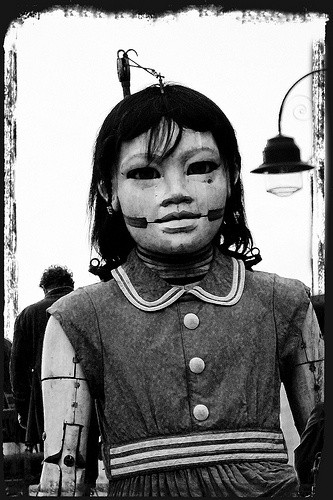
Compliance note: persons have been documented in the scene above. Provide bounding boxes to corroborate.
[8,266,78,453]
[36,81,325,499]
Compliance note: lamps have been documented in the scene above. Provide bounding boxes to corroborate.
[248,67,324,199]
